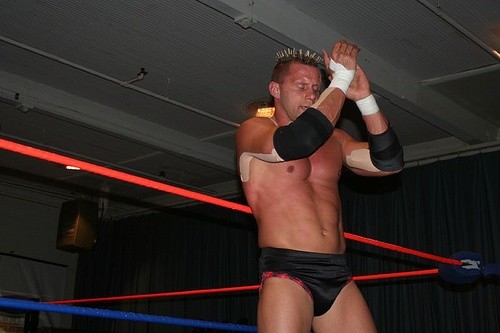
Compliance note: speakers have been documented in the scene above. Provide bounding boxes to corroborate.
[57,200,96,254]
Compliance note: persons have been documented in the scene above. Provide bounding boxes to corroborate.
[233,39,407,332]
